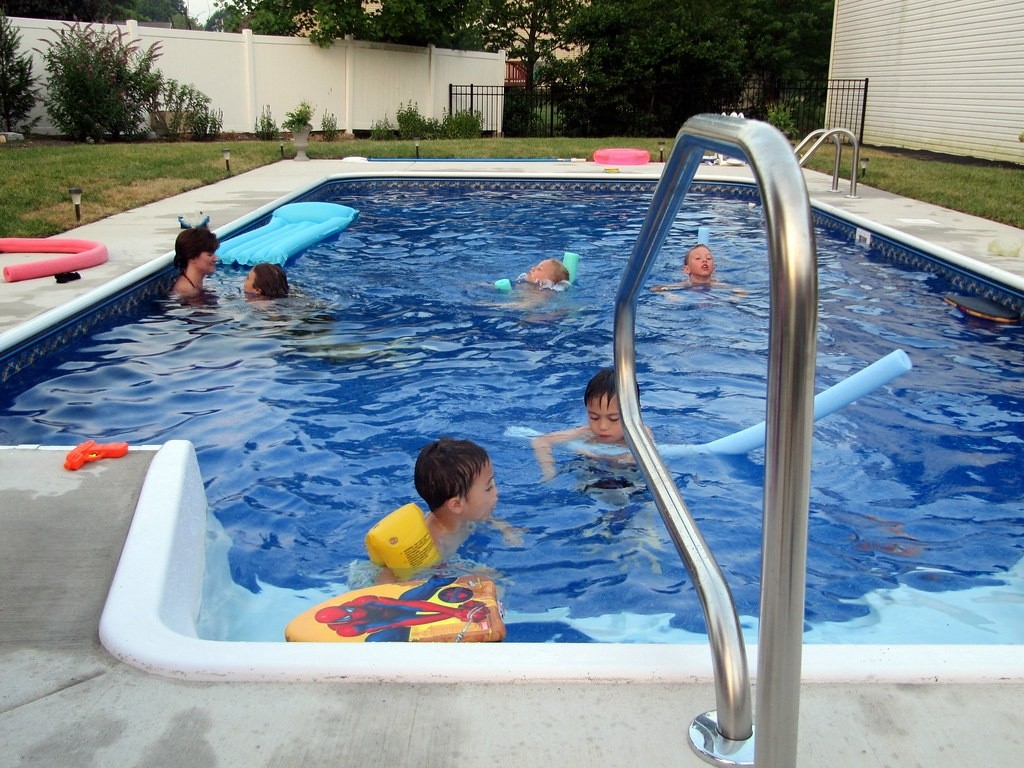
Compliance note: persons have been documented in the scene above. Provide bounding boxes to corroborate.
[170,229,220,293]
[473,258,569,310]
[531,368,655,483]
[244,261,290,295]
[376,439,524,587]
[653,244,748,305]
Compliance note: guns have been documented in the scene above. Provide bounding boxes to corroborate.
[64,440,128,471]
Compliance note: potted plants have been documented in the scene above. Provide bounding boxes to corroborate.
[768,103,799,138]
[283,103,315,161]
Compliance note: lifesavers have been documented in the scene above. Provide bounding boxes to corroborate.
[367,502,442,568]
[593,148,650,165]
[495,248,580,291]
[0,237,109,282]
[714,349,912,453]
[697,226,711,247]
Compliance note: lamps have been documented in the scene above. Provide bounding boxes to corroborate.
[278,137,283,146]
[221,149,231,160]
[860,157,869,168]
[68,189,82,205]
[658,142,665,151]
[414,137,420,146]
